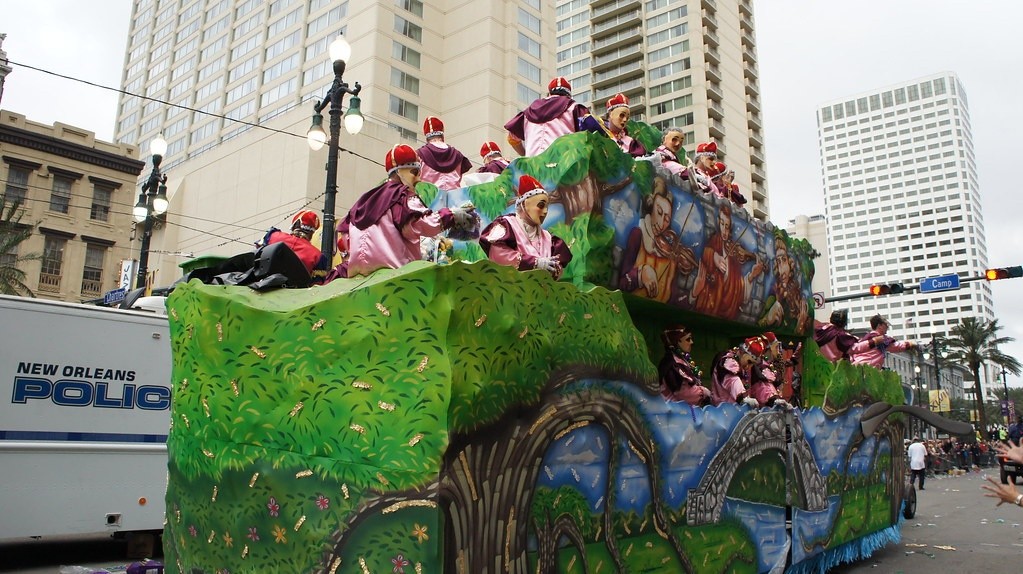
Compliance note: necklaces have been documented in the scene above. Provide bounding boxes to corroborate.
[676,350,702,377]
[764,355,778,376]
[732,352,750,390]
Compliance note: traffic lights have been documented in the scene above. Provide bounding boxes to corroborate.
[870,282,904,296]
[984,265,1023,281]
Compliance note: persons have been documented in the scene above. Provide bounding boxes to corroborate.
[848,313,913,370]
[904,439,911,456]
[920,437,969,476]
[964,439,996,469]
[980,416,1023,509]
[479,174,574,282]
[658,322,711,406]
[714,162,748,205]
[338,142,477,277]
[816,307,862,361]
[908,436,927,490]
[415,116,472,191]
[600,92,646,156]
[749,332,784,408]
[504,77,610,158]
[690,141,727,197]
[474,141,511,175]
[711,336,767,408]
[727,168,739,191]
[650,127,708,186]
[255,209,329,286]
[787,338,802,406]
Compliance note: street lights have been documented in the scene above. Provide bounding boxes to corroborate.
[914,365,924,441]
[922,333,949,440]
[306,27,366,275]
[132,131,169,297]
[995,365,1010,425]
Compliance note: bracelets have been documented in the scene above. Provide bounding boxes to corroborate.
[1015,493,1022,506]
